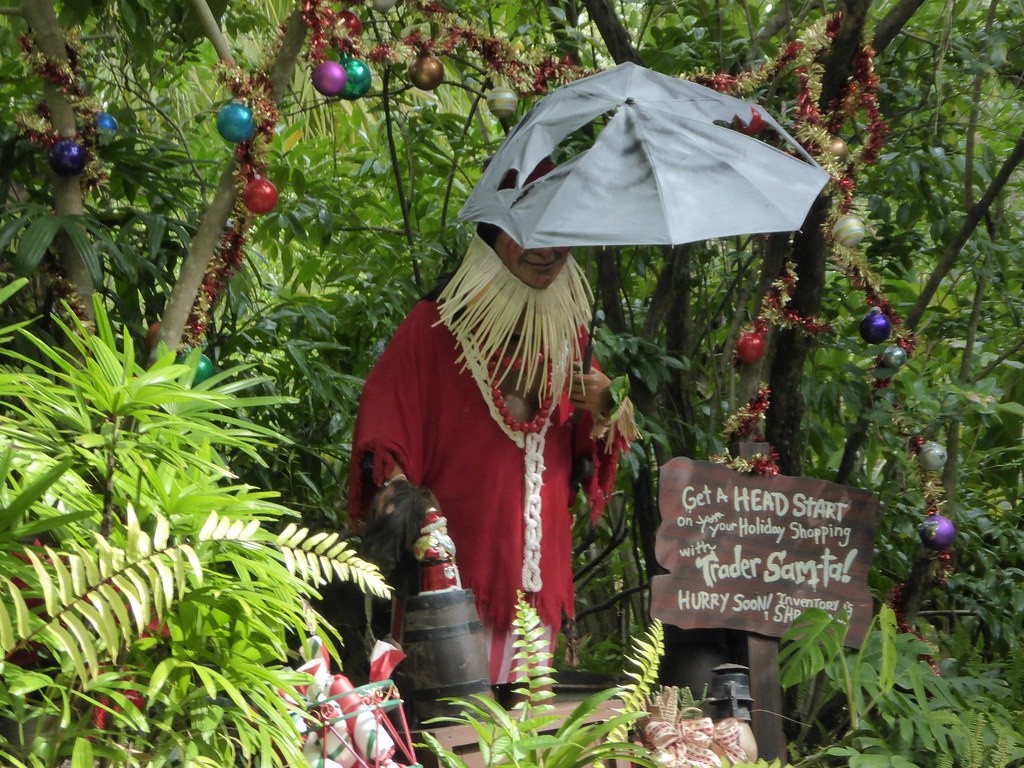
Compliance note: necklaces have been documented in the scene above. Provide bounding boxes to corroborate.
[468,325,553,432]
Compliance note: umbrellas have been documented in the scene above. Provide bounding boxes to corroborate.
[457,62,829,423]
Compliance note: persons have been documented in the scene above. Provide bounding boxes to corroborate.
[346,155,635,690]
